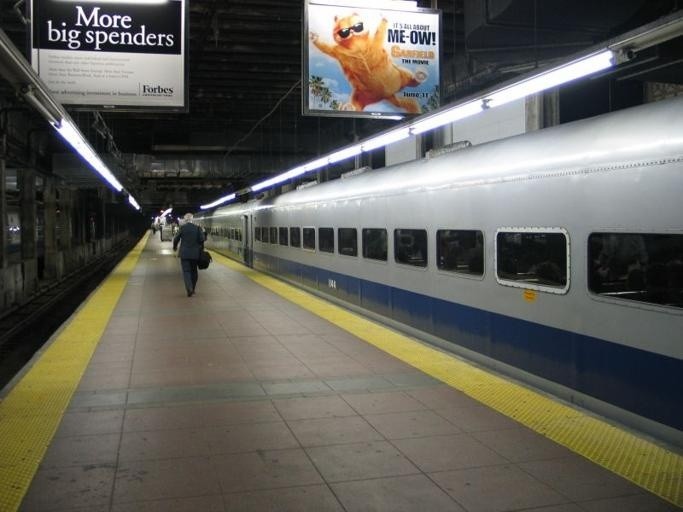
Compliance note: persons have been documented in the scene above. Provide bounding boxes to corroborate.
[173,213,205,296]
[588,234,682,306]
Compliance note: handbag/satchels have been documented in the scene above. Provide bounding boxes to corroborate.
[196,248,212,270]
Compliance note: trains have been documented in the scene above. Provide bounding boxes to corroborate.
[182,97,682,456]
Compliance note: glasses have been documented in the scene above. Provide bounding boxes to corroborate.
[337,23,363,38]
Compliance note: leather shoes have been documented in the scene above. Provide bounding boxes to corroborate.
[187,288,192,296]
